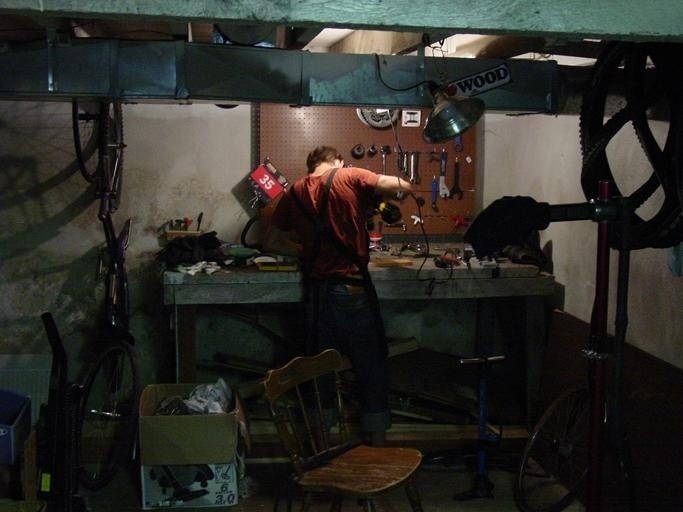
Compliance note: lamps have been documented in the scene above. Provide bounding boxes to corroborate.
[373,51,484,145]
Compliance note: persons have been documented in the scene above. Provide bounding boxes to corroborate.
[271,147,414,449]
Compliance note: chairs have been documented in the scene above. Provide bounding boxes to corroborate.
[259,349,423,512]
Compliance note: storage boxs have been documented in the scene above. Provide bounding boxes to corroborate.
[133,383,247,512]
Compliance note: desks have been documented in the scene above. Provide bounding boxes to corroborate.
[163,241,555,436]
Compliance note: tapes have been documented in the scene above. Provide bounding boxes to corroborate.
[352,144,366,159]
